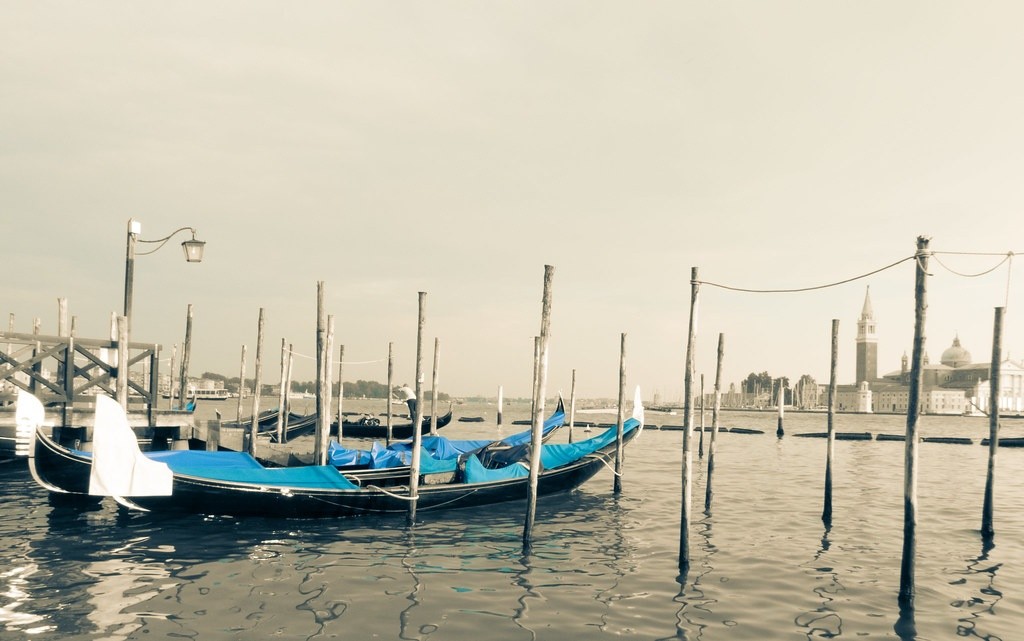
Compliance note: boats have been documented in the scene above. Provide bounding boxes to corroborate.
[31,394,565,495]
[643,406,677,415]
[211,406,317,445]
[162,388,229,401]
[96,385,644,517]
[328,403,453,440]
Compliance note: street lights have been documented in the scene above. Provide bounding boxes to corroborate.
[124,217,206,411]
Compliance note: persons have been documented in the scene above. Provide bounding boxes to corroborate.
[394,383,416,423]
